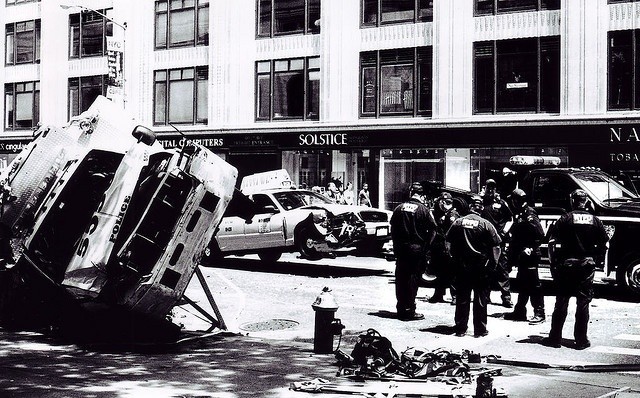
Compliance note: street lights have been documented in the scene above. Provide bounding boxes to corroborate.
[59,4,127,109]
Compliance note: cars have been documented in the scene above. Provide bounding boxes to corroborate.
[201,188,394,266]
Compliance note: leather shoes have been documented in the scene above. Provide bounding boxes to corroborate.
[528,316,546,325]
[504,312,527,320]
[450,325,468,334]
[406,311,425,320]
[428,294,443,303]
[503,300,514,308]
[474,329,489,337]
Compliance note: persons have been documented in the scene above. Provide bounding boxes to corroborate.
[326,178,341,204]
[342,182,356,207]
[428,190,455,304]
[391,185,436,312]
[475,178,514,308]
[444,196,500,314]
[546,190,607,323]
[356,180,374,208]
[505,189,543,322]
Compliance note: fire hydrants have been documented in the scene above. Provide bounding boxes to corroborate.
[311,286,344,354]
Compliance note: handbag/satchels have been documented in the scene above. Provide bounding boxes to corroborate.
[447,260,465,291]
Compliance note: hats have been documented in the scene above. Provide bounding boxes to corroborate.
[471,195,483,205]
[432,194,452,203]
[507,188,526,199]
[486,178,496,184]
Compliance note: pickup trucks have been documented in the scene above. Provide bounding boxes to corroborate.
[420,155,640,297]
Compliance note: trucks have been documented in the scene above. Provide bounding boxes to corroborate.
[1,96,238,337]
